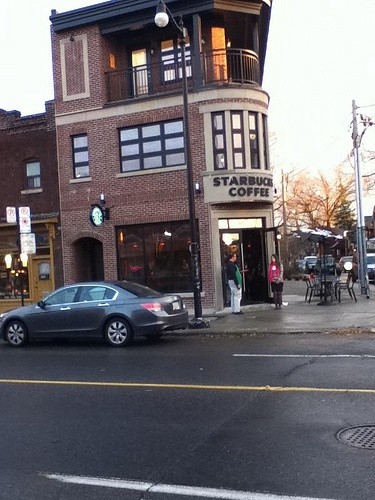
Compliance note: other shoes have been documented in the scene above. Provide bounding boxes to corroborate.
[232,311,244,315]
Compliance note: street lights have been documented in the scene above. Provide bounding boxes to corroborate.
[4,253,29,306]
[348,98,370,295]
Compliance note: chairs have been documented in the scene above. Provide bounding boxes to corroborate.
[303,271,358,304]
[79,290,93,301]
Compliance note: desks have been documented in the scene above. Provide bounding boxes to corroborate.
[316,278,340,307]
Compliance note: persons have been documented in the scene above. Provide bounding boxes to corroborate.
[268,254,284,310]
[226,252,245,316]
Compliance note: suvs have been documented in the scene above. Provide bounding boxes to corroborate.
[351,253,375,285]
[339,256,354,273]
[295,254,335,275]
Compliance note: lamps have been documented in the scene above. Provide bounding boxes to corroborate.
[100,190,107,205]
[195,182,201,194]
[276,228,282,240]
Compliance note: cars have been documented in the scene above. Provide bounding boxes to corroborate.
[0,281,188,348]
